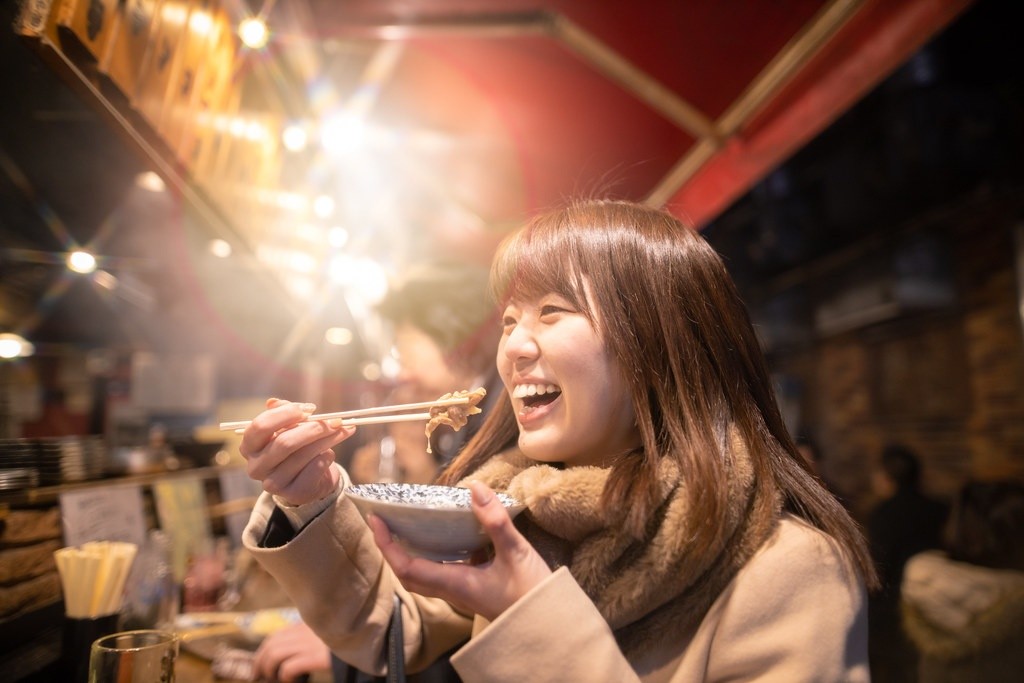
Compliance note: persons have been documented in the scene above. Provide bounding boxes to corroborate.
[237,199,879,683]
[179,264,499,683]
[789,436,1024,683]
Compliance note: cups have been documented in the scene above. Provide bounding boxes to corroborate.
[60,613,183,683]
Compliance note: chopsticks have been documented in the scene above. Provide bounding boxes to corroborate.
[220,396,467,435]
[54,541,137,618]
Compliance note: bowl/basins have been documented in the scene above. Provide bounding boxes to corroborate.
[112,446,161,473]
[345,483,528,562]
[173,443,223,468]
[167,609,303,661]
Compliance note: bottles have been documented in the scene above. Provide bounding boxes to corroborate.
[118,529,174,631]
[182,535,217,613]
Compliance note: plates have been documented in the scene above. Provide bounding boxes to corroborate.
[0,431,114,491]
